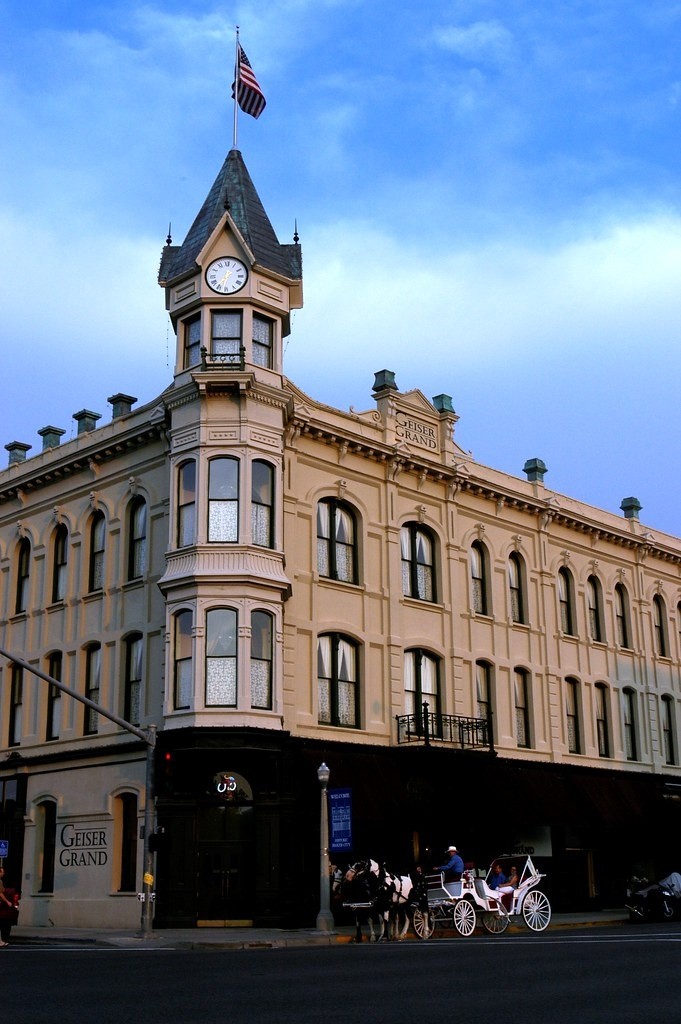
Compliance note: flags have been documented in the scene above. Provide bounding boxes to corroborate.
[327,787,353,852]
[231,41,267,121]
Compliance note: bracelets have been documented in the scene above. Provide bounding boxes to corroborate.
[504,884,505,886]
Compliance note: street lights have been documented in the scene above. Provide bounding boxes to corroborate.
[316,762,335,931]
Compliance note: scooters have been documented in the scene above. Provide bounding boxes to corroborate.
[628,880,676,921]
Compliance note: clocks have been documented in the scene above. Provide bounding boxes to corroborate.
[204,256,249,296]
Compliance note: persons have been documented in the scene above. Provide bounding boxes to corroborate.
[332,865,343,894]
[0,867,13,947]
[488,863,519,897]
[433,845,464,884]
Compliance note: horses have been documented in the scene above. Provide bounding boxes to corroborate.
[331,856,429,942]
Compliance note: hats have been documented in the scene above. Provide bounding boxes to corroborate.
[446,846,458,852]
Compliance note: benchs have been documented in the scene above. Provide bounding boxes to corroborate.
[444,862,474,896]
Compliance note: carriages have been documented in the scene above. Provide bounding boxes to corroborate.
[332,852,552,945]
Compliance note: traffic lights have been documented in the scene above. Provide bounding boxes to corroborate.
[155,741,175,781]
